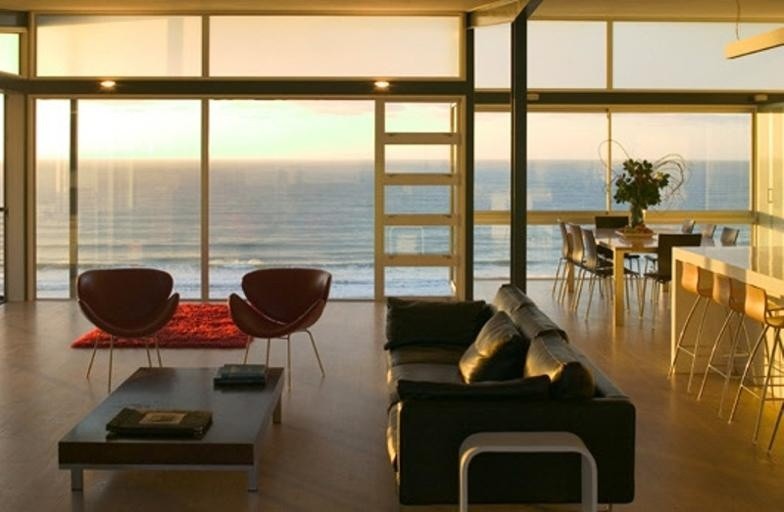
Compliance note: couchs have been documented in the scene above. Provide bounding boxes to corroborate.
[381,281,637,510]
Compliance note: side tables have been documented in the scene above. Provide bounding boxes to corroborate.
[458,433,598,509]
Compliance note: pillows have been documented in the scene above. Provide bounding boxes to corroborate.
[394,374,549,400]
[456,310,526,383]
[383,296,488,350]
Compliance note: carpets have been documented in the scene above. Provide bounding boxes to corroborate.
[70,302,254,349]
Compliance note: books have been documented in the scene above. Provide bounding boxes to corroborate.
[106,408,211,436]
[213,365,269,386]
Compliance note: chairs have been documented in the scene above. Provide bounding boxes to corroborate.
[229,265,332,391]
[76,268,179,393]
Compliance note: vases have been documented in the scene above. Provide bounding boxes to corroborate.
[598,138,688,209]
[631,207,644,224]
